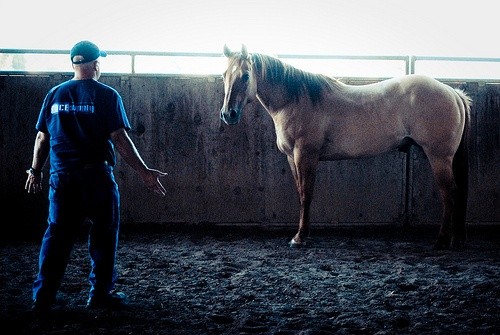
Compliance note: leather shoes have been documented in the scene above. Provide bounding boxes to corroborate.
[29,299,53,312]
[86,290,127,310]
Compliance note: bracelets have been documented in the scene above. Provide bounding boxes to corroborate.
[28,167,42,175]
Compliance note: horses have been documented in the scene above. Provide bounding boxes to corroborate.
[220,43,473,251]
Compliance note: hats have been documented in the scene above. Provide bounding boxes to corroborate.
[69,40,107,66]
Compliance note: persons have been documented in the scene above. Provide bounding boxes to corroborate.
[24,39,168,312]
